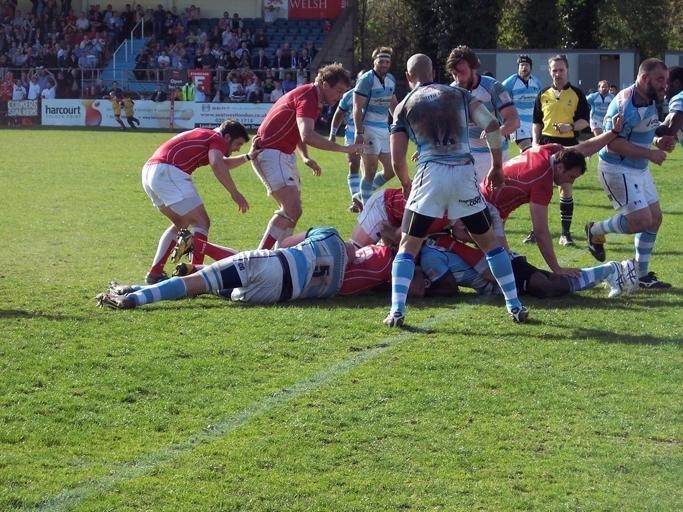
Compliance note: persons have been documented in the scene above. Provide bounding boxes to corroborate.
[103,43,683,329]
[323,19,332,33]
[2,1,318,103]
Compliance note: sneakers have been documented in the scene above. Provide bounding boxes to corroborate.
[585,221,605,262]
[559,234,575,246]
[350,193,363,212]
[382,311,405,327]
[96,280,136,310]
[524,230,536,243]
[145,272,169,284]
[172,262,193,277]
[171,228,193,263]
[512,306,529,323]
[605,258,640,298]
[639,271,671,289]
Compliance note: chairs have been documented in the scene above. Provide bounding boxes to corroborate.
[184,16,336,67]
